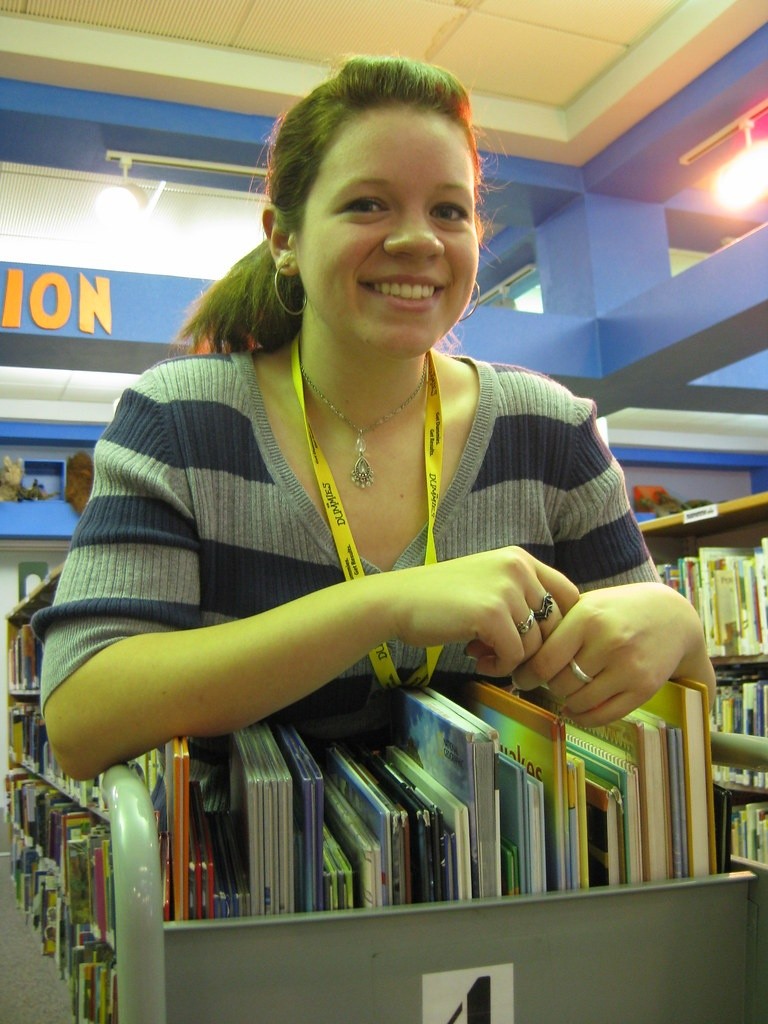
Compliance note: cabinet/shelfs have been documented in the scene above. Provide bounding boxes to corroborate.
[4,561,163,1023]
[637,491,768,868]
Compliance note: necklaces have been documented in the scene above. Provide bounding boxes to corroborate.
[301,358,428,489]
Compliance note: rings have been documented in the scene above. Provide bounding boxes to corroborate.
[534,594,553,621]
[570,659,593,684]
[517,609,536,635]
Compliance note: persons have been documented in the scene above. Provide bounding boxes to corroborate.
[33,56,717,780]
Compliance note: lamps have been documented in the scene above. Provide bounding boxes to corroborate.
[93,155,147,219]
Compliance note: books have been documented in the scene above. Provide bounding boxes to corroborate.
[653,536,768,660]
[709,672,768,873]
[171,680,718,921]
[8,623,44,689]
[10,704,115,1024]
